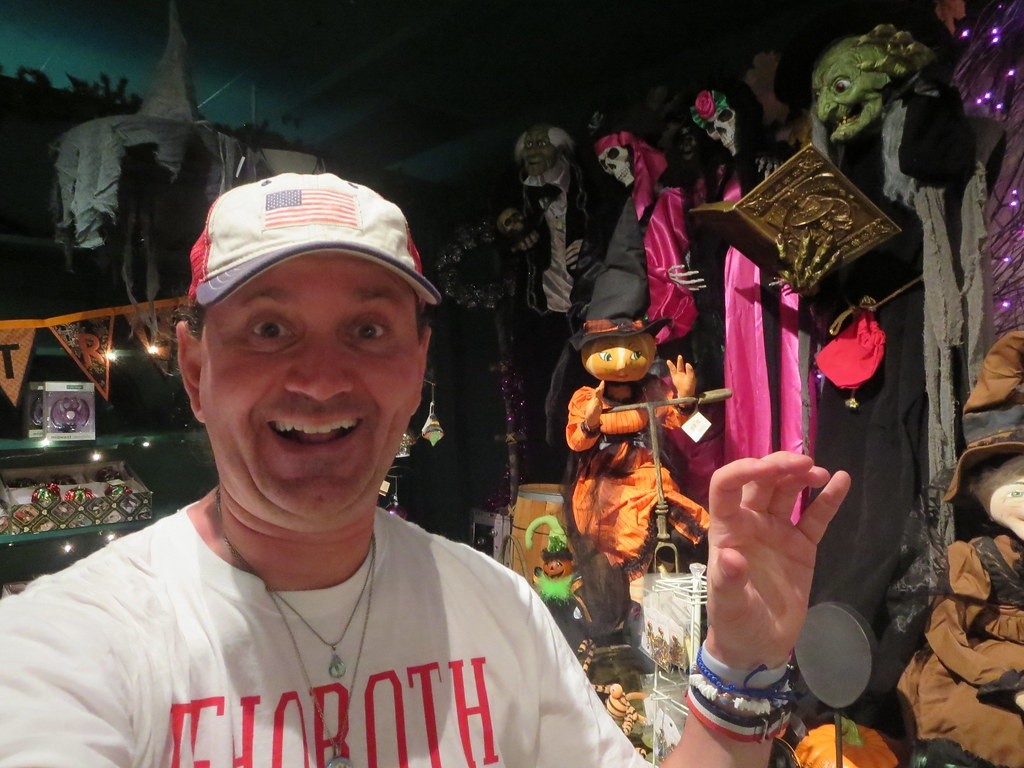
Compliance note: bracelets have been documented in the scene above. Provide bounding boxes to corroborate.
[684,639,794,743]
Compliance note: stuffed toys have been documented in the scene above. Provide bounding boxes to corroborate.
[896,332,1024,768]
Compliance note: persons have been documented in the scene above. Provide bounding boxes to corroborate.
[564,198,710,582]
[0,171,853,768]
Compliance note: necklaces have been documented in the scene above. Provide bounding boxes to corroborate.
[216,486,375,768]
[217,487,375,677]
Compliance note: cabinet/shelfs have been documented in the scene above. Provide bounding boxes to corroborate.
[0,346,207,543]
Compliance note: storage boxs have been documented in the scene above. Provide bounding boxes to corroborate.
[41,382,96,441]
[0,460,153,534]
[22,380,43,439]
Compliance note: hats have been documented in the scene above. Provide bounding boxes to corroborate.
[188,172,442,308]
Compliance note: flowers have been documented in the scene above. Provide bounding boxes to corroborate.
[694,90,716,119]
